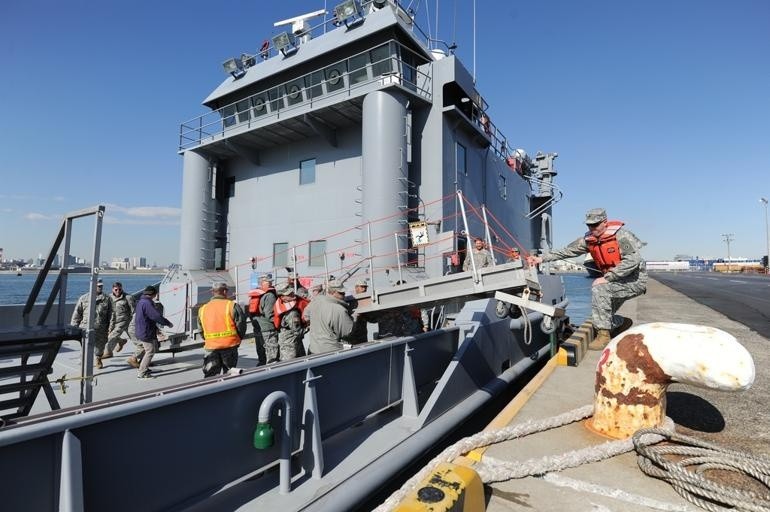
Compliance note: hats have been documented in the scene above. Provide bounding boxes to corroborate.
[97,278,103,287]
[143,286,157,295]
[585,208,607,224]
[213,272,369,296]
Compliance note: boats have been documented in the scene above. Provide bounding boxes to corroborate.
[583,253,603,277]
[16,273,22,277]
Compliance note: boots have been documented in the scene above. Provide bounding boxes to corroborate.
[588,317,632,350]
[96,339,156,380]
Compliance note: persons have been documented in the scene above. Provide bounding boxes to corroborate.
[198,271,429,378]
[70,278,174,378]
[462,237,523,272]
[524,207,649,350]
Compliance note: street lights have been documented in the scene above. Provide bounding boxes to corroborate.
[759,198,770,269]
[721,232,736,273]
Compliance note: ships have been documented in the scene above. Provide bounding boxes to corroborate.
[0,0,572,512]
